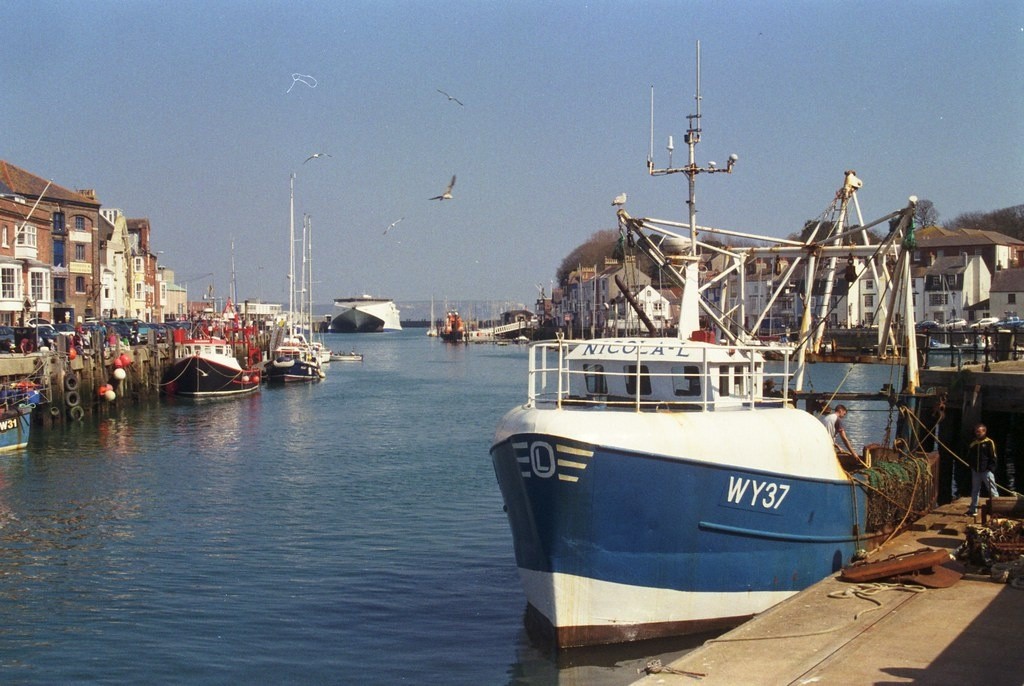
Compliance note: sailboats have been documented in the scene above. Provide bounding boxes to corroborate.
[263,172,331,401]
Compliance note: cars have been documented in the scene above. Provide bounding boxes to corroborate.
[894,313,1024,331]
[0,317,210,350]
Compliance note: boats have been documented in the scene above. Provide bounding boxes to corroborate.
[0,347,53,453]
[425,293,529,346]
[488,38,946,650]
[334,294,403,333]
[333,353,364,362]
[162,341,263,401]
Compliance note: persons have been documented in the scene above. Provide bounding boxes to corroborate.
[241,317,245,326]
[828,319,832,329]
[838,319,847,327]
[823,405,859,461]
[252,318,256,323]
[964,423,1000,516]
[74,322,83,336]
[274,318,284,328]
[0,338,15,353]
[856,320,859,326]
[862,319,864,327]
[99,323,108,347]
[16,337,34,356]
[81,329,91,346]
[132,321,139,345]
[109,333,117,347]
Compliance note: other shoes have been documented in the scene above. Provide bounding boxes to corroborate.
[964,511,978,517]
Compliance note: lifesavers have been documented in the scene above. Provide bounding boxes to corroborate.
[64,373,85,421]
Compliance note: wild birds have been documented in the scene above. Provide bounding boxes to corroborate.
[611,192,628,207]
[383,217,404,235]
[438,88,464,108]
[427,175,456,201]
[303,151,332,163]
